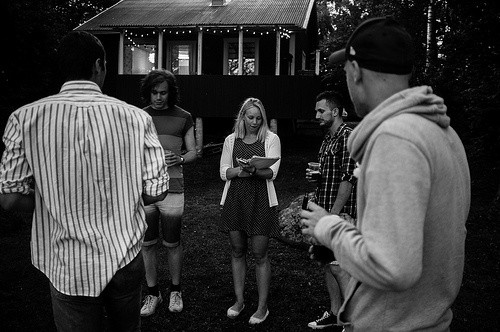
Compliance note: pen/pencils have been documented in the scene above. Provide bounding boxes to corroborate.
[302,192,309,210]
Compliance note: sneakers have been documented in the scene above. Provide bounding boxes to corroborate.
[307,310,342,329]
[140,290,163,317]
[168,290,183,312]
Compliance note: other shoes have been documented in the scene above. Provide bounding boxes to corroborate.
[226,302,245,318]
[248,308,269,324]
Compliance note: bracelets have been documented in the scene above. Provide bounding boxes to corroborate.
[180,155,184,162]
[249,167,256,176]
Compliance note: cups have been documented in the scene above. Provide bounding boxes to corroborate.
[308,161,321,182]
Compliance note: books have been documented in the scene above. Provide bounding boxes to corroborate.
[238,155,279,177]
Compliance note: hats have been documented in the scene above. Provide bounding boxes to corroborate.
[329,14,414,77]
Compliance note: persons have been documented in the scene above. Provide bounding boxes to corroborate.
[0,31,169,332]
[299,14,470,332]
[220,97,281,324]
[306,92,358,332]
[141,70,197,317]
